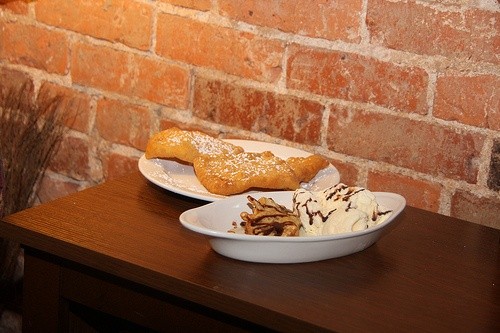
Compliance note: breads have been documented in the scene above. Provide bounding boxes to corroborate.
[287,153,330,184]
[145,126,243,163]
[194,149,300,194]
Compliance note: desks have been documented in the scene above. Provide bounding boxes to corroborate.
[0,159,500,333]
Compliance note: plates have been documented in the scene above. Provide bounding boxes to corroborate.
[179,190,407,263]
[138,138,341,202]
